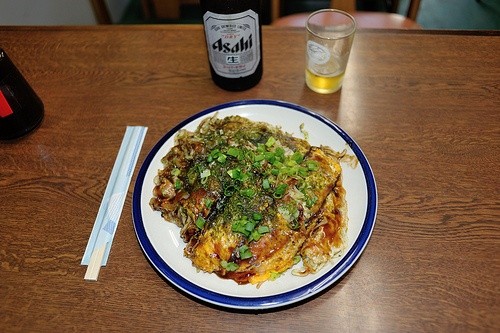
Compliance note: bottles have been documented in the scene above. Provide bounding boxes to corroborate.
[201,0,263,91]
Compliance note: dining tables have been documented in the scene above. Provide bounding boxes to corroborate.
[0,24,500,332]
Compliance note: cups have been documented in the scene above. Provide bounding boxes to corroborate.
[304,9,356,95]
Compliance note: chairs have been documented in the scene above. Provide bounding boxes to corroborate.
[271,0,421,26]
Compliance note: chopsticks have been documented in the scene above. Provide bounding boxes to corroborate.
[84,126,141,280]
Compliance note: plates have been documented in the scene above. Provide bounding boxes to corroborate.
[131,100,379,309]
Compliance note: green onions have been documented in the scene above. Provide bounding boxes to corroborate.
[178,128,320,259]
